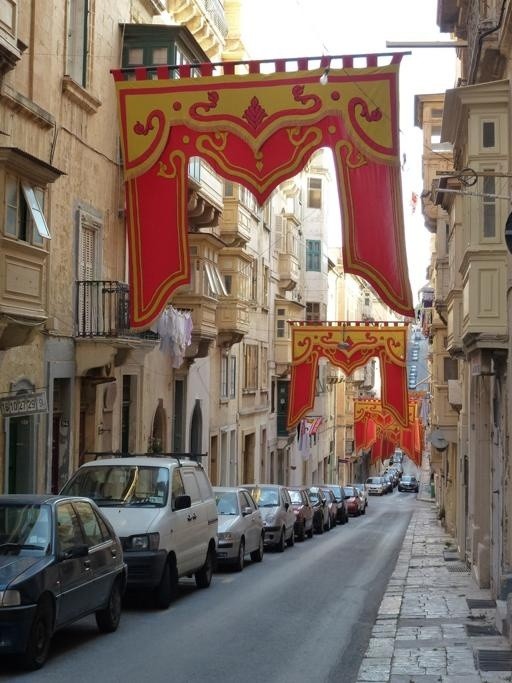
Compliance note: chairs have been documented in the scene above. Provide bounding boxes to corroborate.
[100,468,122,499]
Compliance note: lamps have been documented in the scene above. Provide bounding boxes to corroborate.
[98,422,112,435]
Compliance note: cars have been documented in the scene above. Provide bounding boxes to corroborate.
[364,451,419,496]
[0,489,131,675]
[210,482,368,575]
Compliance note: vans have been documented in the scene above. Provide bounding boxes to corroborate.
[52,450,221,616]
